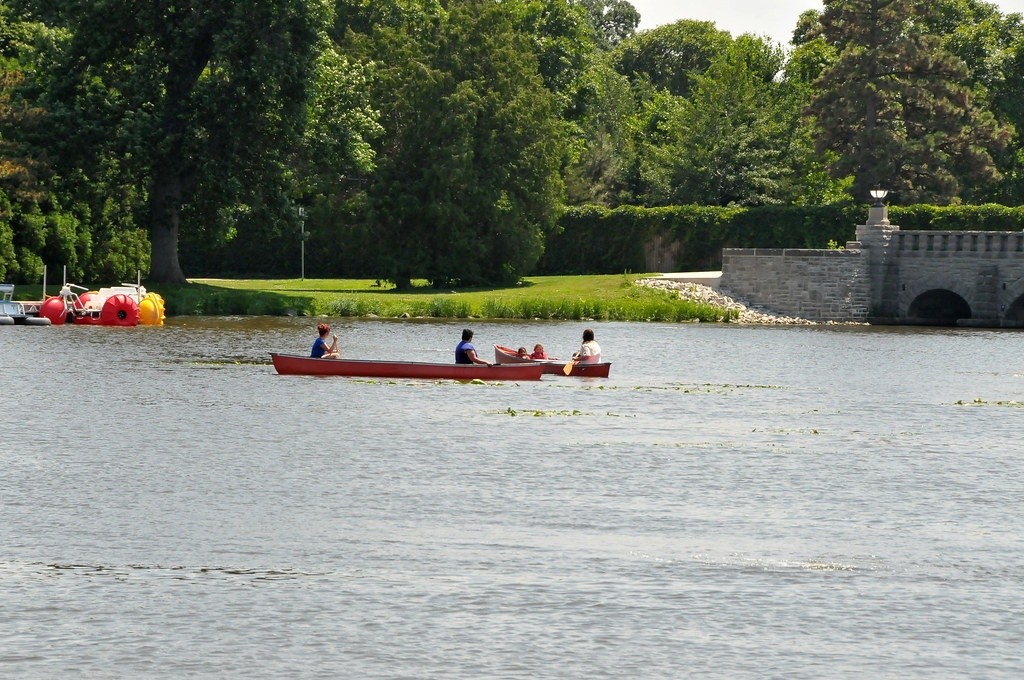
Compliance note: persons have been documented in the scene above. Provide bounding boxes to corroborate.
[454,329,492,368]
[515,347,529,359]
[530,343,558,361]
[572,329,601,364]
[310,324,340,359]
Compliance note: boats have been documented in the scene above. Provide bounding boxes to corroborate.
[268,352,547,380]
[492,344,611,378]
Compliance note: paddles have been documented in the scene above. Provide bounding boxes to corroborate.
[562,351,581,376]
[333,334,339,353]
